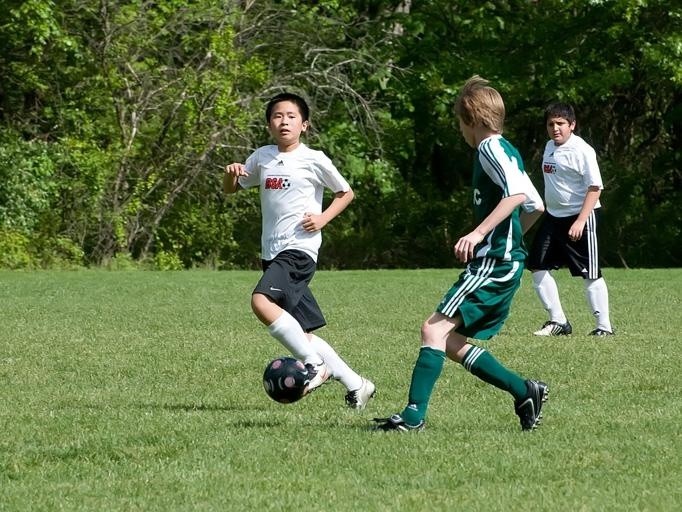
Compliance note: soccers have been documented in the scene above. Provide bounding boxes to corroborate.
[262,358,308,404]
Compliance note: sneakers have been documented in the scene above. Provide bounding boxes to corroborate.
[534,320,570,335]
[514,381,549,432]
[346,377,375,410]
[303,360,330,394]
[373,415,423,433]
[587,328,612,337]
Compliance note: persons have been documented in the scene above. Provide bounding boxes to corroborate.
[369,72,553,440]
[216,92,380,409]
[516,101,614,336]
